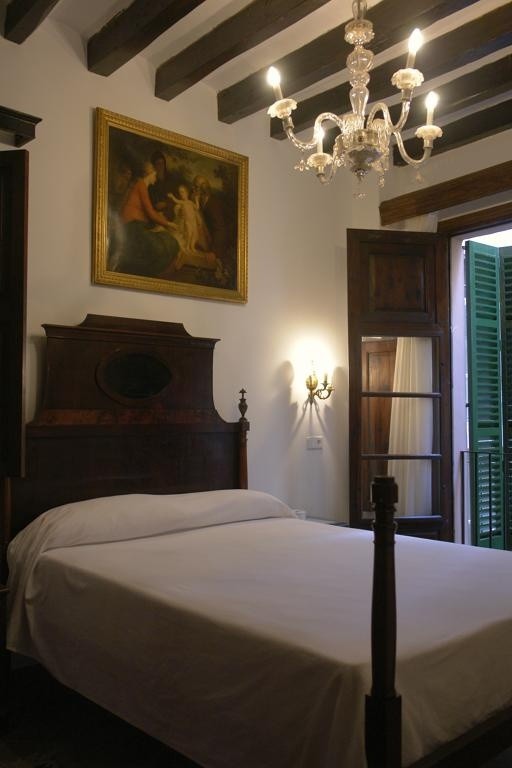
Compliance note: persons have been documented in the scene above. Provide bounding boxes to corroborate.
[118,149,236,278]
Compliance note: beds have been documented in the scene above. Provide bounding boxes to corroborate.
[4,311,509,768]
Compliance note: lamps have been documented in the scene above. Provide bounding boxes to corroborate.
[265,0,444,200]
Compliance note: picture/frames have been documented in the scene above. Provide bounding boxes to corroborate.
[89,105,250,309]
